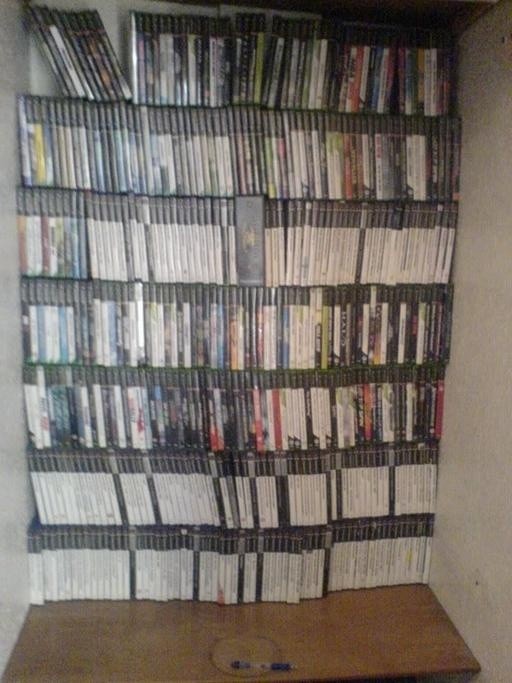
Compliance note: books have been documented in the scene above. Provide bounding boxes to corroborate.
[16,0,462,607]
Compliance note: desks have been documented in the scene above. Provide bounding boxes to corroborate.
[1,584,481,682]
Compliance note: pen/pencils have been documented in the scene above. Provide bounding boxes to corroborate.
[230,661,299,670]
